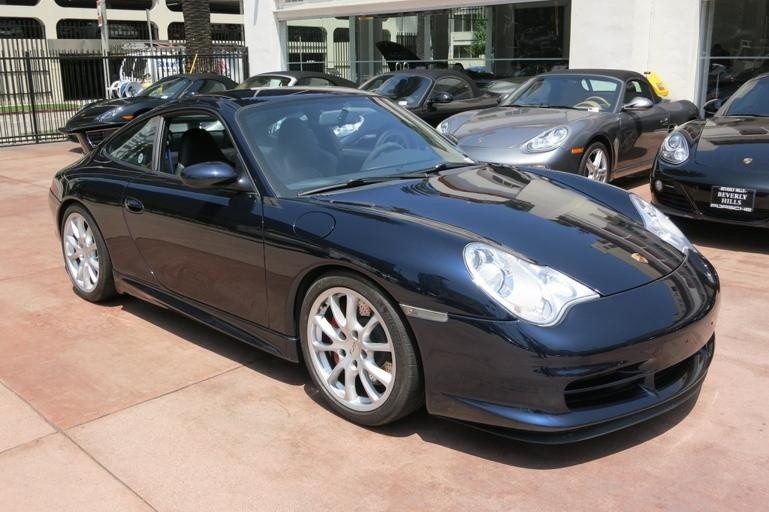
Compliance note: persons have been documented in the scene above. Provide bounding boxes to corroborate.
[455,63,464,72]
[711,43,731,69]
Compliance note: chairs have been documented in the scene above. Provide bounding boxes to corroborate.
[160,82,221,99]
[542,81,643,116]
[171,118,343,193]
